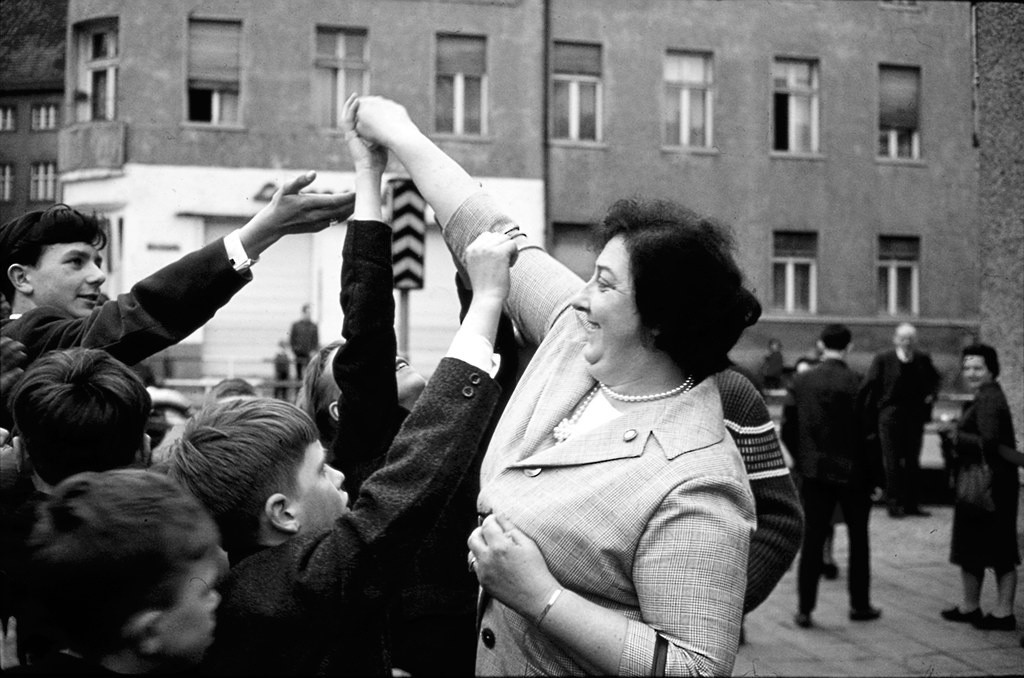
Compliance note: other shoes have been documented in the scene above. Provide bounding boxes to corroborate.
[823,562,838,579]
[849,605,881,621]
[941,606,1016,632]
[795,611,812,627]
[887,508,931,517]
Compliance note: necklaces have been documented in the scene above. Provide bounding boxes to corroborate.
[553,374,695,402]
[551,384,598,442]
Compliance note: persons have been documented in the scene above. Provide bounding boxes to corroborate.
[0,90,1024,678]
[355,95,762,678]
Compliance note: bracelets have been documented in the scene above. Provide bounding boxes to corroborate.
[533,586,565,627]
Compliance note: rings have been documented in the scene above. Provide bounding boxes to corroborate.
[468,557,477,572]
[329,217,339,227]
